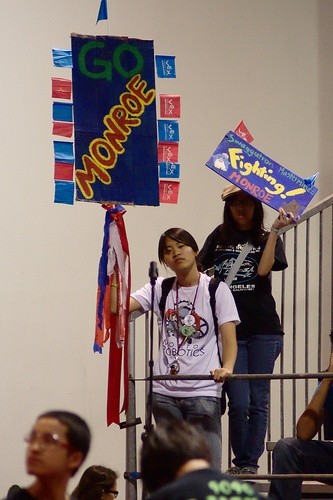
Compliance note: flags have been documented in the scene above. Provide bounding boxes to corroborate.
[96,0,108,25]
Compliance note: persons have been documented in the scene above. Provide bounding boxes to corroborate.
[3,330,333,500]
[109,229,241,473]
[196,183,301,476]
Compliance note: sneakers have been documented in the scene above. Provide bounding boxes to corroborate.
[240,466,257,474]
[226,467,242,474]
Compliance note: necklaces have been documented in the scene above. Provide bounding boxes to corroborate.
[170,273,200,374]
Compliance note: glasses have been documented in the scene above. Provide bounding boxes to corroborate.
[227,198,251,206]
[105,491,118,498]
[24,431,68,448]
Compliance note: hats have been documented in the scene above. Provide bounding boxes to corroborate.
[221,185,243,201]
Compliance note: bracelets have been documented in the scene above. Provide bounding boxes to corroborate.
[110,283,118,287]
[270,228,280,232]
